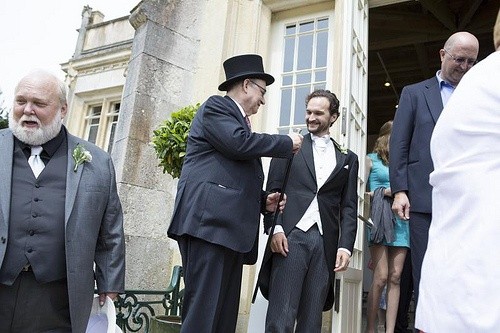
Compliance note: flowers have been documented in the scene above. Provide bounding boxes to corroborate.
[339,144,349,155]
[72,145,92,171]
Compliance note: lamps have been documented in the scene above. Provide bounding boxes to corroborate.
[384,78,391,87]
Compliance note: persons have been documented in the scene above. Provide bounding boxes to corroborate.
[364,121,409,333]
[389,32,479,333]
[258,90,358,333]
[166,54,303,333]
[0,69,126,333]
[414,6,500,333]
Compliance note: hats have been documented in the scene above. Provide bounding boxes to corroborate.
[85,295,122,333]
[218,54,275,91]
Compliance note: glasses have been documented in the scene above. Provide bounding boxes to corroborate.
[250,80,266,97]
[445,50,478,65]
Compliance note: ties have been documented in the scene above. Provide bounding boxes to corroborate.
[28,145,46,180]
[315,139,326,154]
